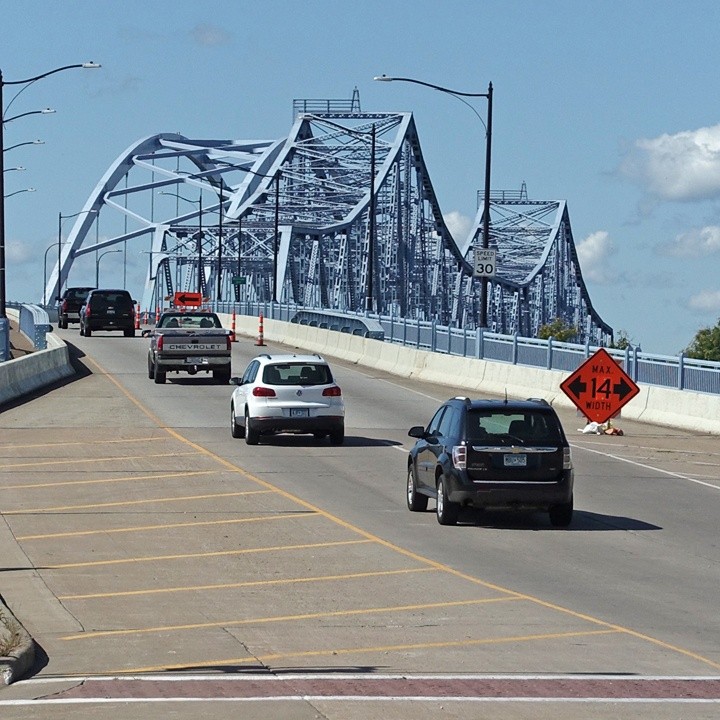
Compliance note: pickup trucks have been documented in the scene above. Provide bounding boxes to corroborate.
[141,309,232,384]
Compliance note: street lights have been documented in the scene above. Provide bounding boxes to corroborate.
[97,249,122,290]
[373,76,493,330]
[158,191,202,293]
[172,170,223,302]
[44,242,73,309]
[299,111,377,314]
[58,210,98,303]
[0,61,103,363]
[217,162,279,305]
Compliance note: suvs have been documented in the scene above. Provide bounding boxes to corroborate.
[406,396,574,528]
[55,288,95,329]
[229,353,346,447]
[79,289,137,337]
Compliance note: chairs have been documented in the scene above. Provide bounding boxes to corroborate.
[166,321,178,328]
[269,369,281,380]
[200,319,213,328]
[468,415,487,438]
[509,420,530,437]
[93,296,102,301]
[116,296,125,302]
[300,367,313,380]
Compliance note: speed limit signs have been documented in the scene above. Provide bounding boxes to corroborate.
[474,249,496,277]
